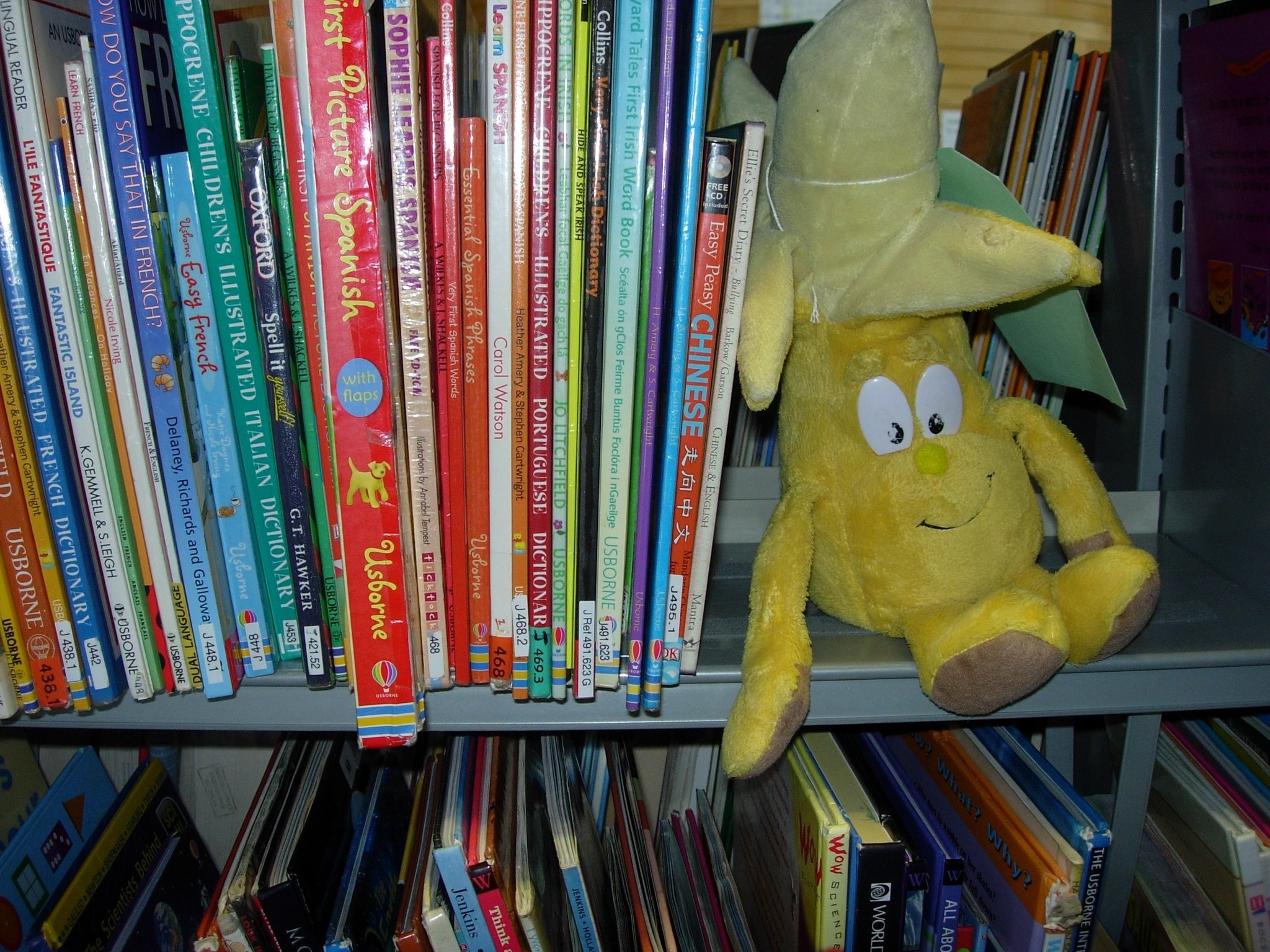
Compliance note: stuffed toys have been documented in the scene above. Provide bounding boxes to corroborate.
[718,0,1162,781]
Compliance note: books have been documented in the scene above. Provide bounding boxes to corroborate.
[2,0,1268,951]
